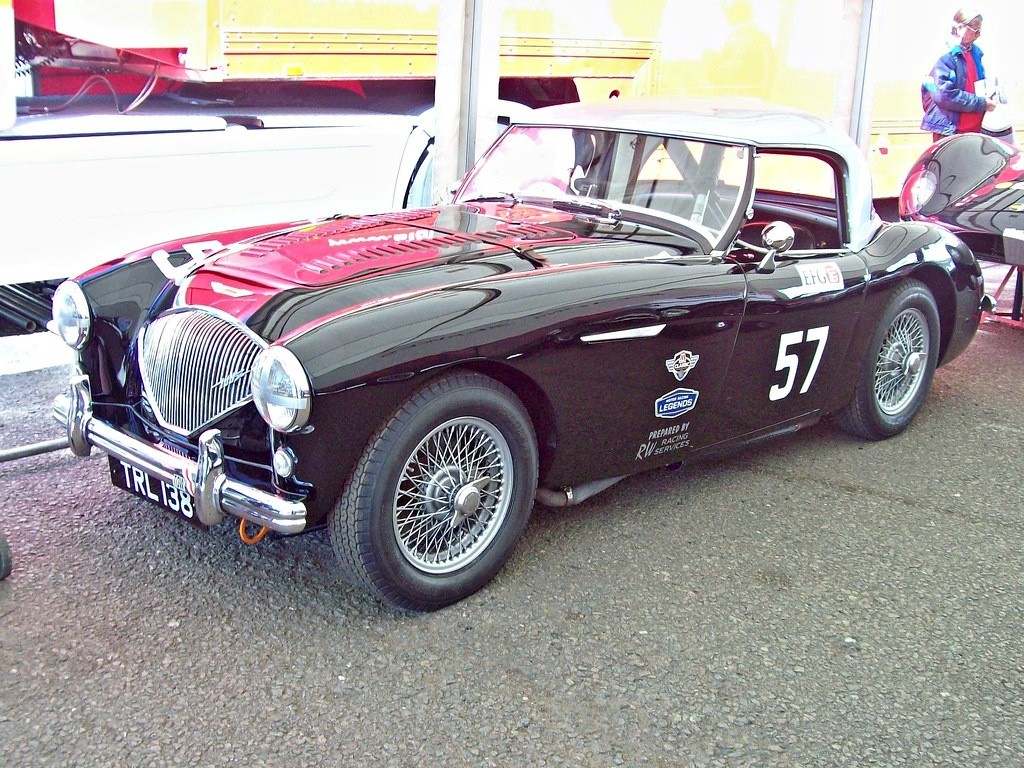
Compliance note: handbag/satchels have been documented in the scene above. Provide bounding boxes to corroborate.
[980,103,1012,144]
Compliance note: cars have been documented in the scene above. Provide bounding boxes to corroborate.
[53,103,998,614]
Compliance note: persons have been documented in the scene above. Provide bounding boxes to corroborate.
[921,9,996,144]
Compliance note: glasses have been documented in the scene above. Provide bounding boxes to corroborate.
[965,25,980,37]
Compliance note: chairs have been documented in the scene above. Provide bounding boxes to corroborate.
[741,221,818,252]
